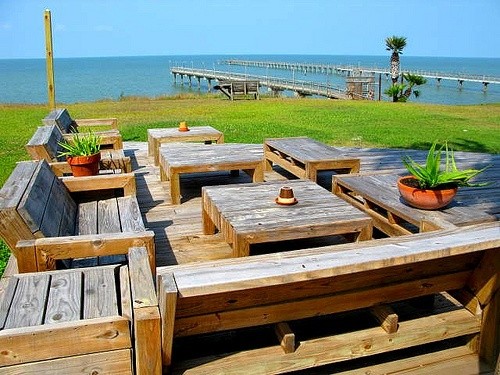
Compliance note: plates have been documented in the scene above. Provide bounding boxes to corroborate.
[178,129,189,132]
[275,198,298,205]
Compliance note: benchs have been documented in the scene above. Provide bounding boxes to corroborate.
[229,80,259,100]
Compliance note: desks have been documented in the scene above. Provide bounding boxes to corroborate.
[147,125,224,165]
[330,170,497,238]
[157,144,266,206]
[264,136,360,184]
[200,179,374,261]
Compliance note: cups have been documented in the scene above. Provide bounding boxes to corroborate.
[277,186,295,203]
[179,122,187,130]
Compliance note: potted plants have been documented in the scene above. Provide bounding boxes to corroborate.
[55,124,101,177]
[398,138,496,210]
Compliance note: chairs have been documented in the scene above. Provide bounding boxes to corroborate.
[0,108,163,375]
